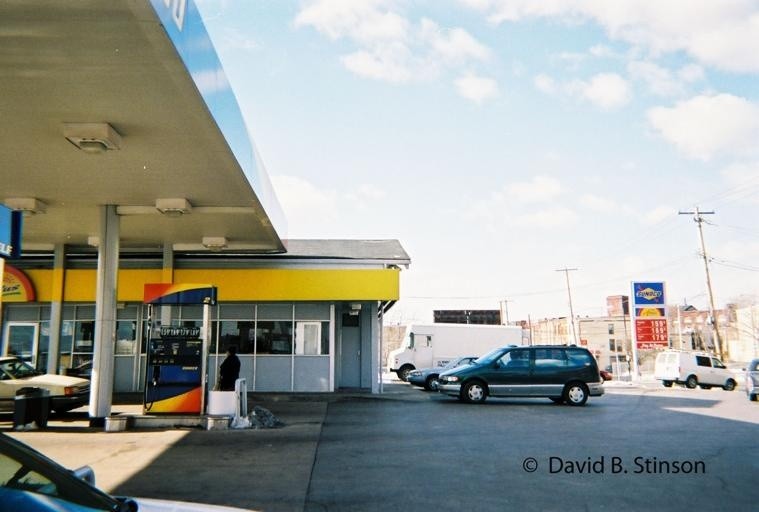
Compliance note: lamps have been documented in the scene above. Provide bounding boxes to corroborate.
[62,122,121,153]
[157,197,193,218]
[201,237,229,250]
[4,199,44,219]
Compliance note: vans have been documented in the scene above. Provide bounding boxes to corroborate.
[654,349,739,391]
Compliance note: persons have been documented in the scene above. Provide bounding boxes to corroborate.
[219,346,240,391]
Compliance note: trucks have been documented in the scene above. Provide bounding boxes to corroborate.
[387,322,523,381]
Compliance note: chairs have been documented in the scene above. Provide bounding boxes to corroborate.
[507,351,523,368]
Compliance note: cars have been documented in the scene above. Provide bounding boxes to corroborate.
[0,356,91,414]
[406,343,605,406]
[1,432,257,512]
[742,357,759,401]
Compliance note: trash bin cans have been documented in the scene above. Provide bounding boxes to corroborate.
[13,387,50,430]
